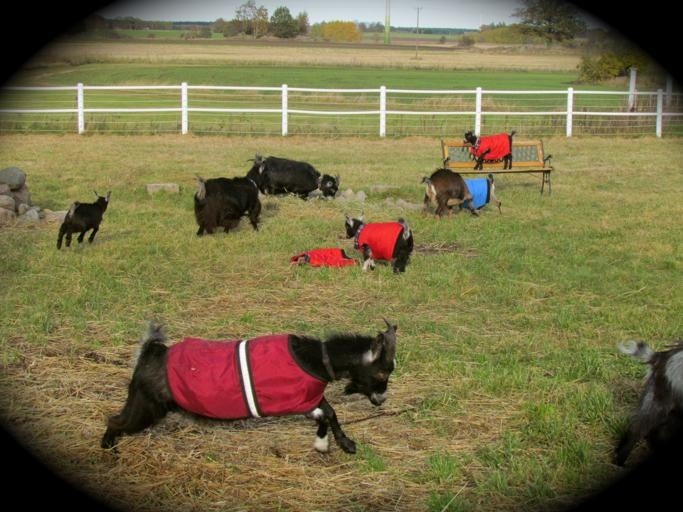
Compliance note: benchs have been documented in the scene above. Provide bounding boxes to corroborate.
[441,140,553,195]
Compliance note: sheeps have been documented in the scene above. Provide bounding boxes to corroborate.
[421,166,481,222]
[99,316,399,455]
[180,170,262,235]
[290,247,360,267]
[245,153,340,203]
[535,336,683,511]
[56,189,113,250]
[344,210,414,275]
[462,128,516,170]
[458,173,504,216]
